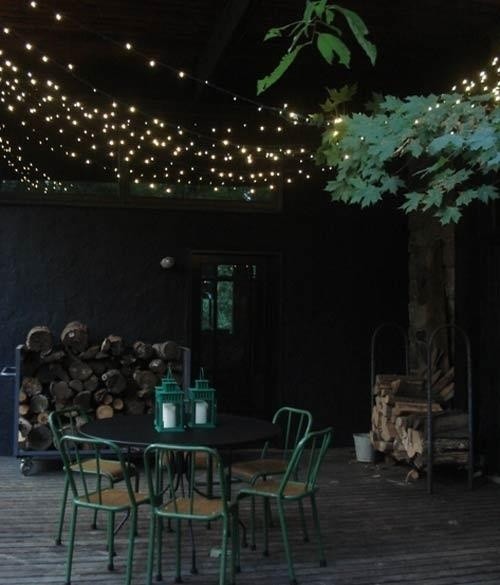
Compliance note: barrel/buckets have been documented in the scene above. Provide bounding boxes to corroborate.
[353,432,375,463]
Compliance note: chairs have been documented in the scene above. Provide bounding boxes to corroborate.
[49,412,139,549]
[143,444,235,584]
[57,435,158,583]
[235,427,334,584]
[226,408,313,546]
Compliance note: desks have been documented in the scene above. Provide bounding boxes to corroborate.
[78,416,253,532]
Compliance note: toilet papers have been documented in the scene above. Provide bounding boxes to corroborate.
[161,403,174,421]
[164,406,177,429]
[195,402,209,424]
